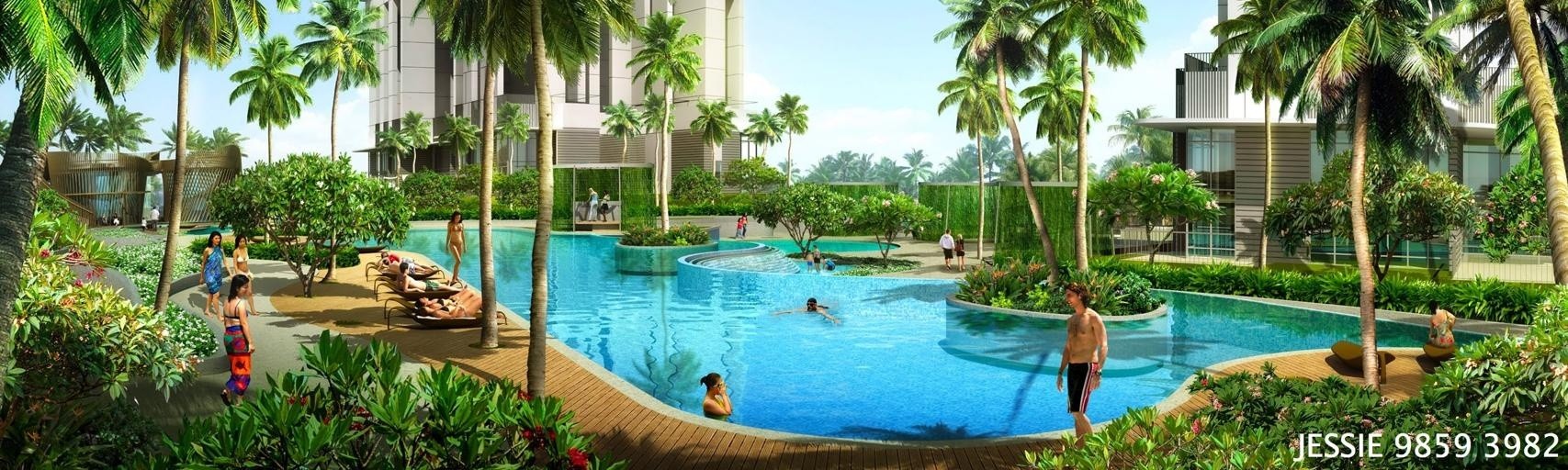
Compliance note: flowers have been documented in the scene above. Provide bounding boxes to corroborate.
[955,253,1168,316]
[620,218,712,246]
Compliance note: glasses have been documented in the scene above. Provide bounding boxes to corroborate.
[712,382,723,387]
[807,303,815,307]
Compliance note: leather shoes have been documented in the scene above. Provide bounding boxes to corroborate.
[220,392,231,406]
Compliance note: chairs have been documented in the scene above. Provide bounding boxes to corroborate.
[1331,340,1455,385]
[366,263,507,332]
